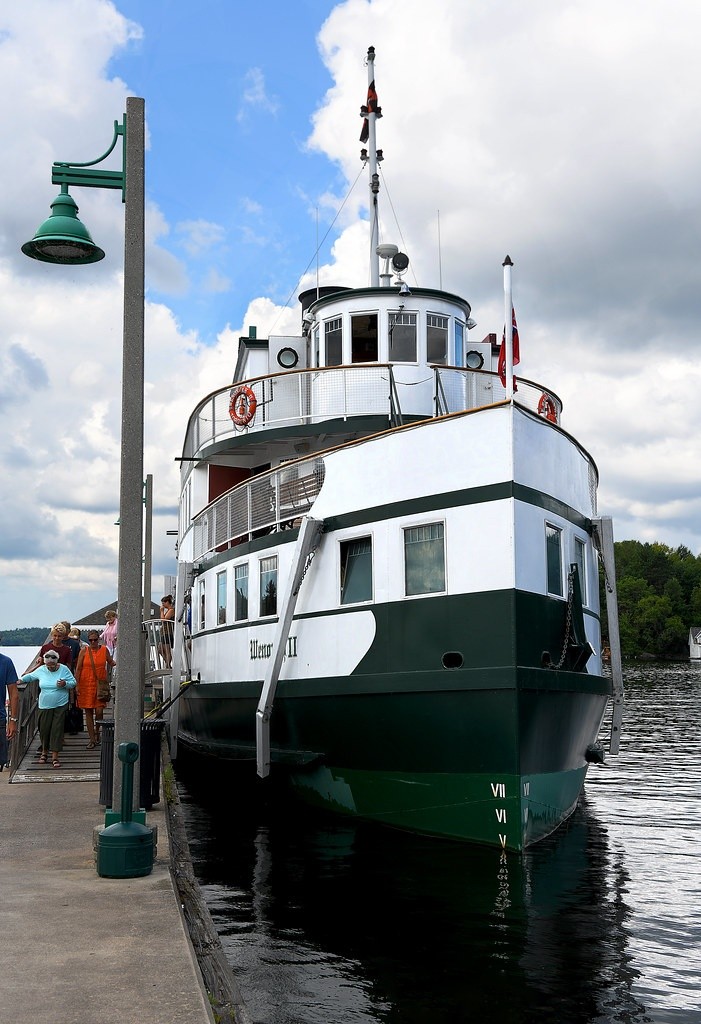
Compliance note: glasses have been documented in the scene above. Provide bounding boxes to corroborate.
[45,654,57,659]
[88,638,98,642]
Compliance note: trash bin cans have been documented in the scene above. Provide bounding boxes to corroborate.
[95,718,166,811]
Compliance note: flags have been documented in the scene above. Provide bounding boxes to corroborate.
[498,301,520,391]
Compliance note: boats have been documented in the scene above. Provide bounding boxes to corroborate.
[144,44,628,852]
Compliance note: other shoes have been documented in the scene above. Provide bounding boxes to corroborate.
[36,746,42,754]
[95,733,101,744]
[86,742,96,749]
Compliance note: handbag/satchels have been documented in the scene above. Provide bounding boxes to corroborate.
[97,680,112,702]
[64,701,84,733]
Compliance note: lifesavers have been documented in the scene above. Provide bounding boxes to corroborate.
[538,393,556,423]
[229,386,257,425]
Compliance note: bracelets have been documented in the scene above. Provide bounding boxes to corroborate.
[10,717,18,722]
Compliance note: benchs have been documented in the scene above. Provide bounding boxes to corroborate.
[227,470,325,542]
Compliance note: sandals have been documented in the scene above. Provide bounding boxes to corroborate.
[52,759,61,767]
[40,755,48,764]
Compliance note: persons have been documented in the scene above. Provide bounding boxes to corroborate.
[99,610,117,687]
[0,652,19,772]
[74,630,117,748]
[21,621,89,735]
[17,649,78,767]
[158,595,175,669]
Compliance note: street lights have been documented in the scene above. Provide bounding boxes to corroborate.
[21,93,169,876]
